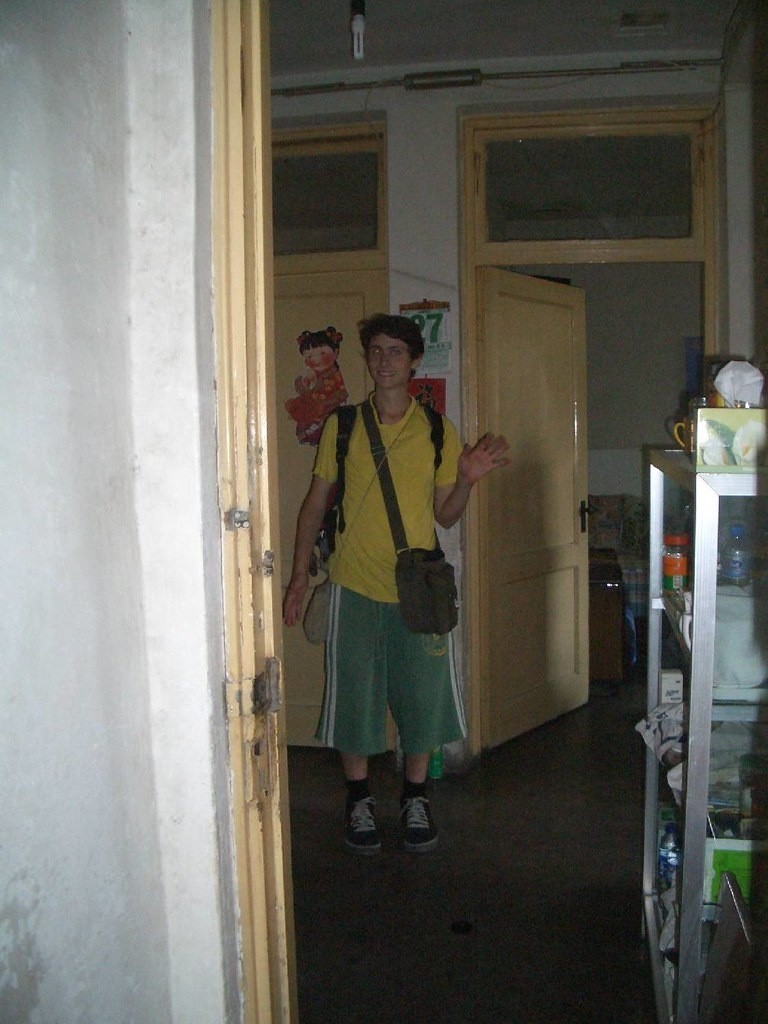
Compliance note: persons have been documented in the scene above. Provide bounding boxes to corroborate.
[283,314,514,854]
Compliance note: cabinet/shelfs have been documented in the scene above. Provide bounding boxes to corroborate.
[634,450,768,1024]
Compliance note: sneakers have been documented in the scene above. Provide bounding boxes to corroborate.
[398,794,441,852]
[344,795,384,848]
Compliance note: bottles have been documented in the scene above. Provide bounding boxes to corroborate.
[720,526,755,589]
[684,396,708,455]
[663,531,690,600]
[655,810,686,892]
[426,745,445,779]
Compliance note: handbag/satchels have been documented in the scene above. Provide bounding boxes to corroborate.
[394,548,460,634]
[304,582,331,646]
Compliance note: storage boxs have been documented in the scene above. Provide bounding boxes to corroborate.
[657,669,684,738]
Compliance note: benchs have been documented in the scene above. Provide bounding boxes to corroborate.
[587,548,625,687]
